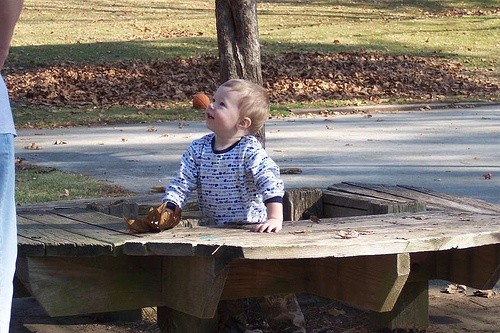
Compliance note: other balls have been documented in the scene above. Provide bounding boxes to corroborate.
[193,94,209,109]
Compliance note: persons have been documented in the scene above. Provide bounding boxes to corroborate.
[164,78,307,333]
[0,0,27,333]
